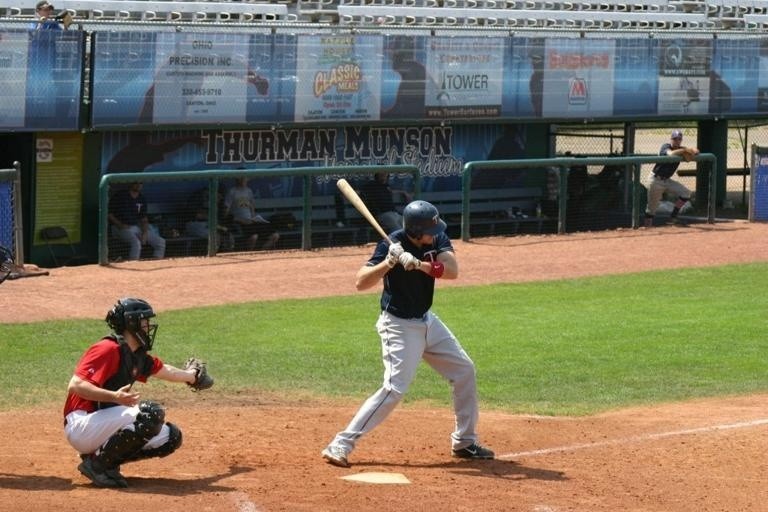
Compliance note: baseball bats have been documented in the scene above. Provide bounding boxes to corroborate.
[336,177,416,271]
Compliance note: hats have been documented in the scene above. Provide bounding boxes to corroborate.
[671,130,683,139]
[36,0,54,11]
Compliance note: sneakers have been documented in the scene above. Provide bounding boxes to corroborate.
[322,445,349,466]
[452,444,495,459]
[77,457,128,488]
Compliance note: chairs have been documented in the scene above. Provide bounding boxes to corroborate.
[0,0,767,37]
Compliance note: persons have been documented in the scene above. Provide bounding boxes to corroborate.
[332,186,346,228]
[354,36,450,118]
[524,40,587,119]
[105,129,205,176]
[0,257,50,281]
[139,39,269,120]
[222,168,280,251]
[63,295,213,487]
[674,39,730,112]
[551,149,648,218]
[185,177,225,254]
[338,164,414,232]
[109,183,167,260]
[646,130,700,226]
[323,199,496,467]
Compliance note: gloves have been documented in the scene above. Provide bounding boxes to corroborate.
[386,241,403,268]
[399,252,421,271]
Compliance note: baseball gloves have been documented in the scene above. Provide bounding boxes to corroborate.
[186,358,214,389]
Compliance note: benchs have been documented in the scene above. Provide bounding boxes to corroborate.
[110,183,549,254]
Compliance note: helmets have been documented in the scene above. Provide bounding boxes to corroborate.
[402,200,448,240]
[107,298,157,351]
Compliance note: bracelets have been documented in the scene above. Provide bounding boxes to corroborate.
[427,261,444,279]
